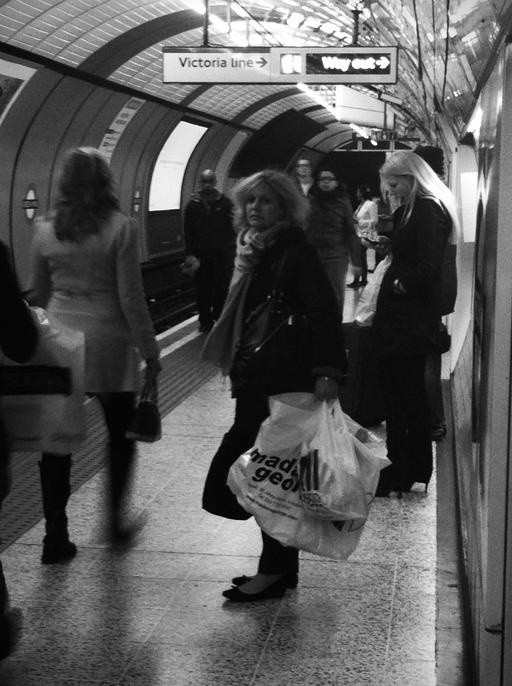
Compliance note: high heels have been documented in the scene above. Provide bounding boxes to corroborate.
[372,462,434,498]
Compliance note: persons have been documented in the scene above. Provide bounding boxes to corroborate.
[0,237,47,661]
[184,170,238,334]
[295,157,367,282]
[24,140,166,569]
[197,167,347,610]
[362,151,457,499]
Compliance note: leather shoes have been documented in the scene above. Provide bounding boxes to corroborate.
[221,581,288,603]
[229,573,300,590]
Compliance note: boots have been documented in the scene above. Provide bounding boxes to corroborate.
[103,441,149,552]
[38,460,78,565]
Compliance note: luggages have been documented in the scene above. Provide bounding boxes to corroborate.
[337,321,387,430]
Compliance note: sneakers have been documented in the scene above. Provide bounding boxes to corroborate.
[347,279,368,290]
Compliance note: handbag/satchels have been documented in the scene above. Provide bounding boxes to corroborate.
[203,424,265,522]
[127,401,162,445]
[0,363,82,456]
[405,242,459,319]
[229,296,287,385]
